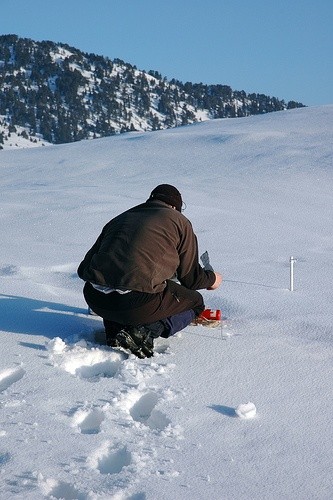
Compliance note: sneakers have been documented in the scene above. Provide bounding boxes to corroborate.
[106,326,155,359]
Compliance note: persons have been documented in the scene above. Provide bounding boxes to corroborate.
[76,183,222,359]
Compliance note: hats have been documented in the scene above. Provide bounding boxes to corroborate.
[150,184,182,213]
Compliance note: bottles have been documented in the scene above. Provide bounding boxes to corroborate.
[200,309,221,320]
